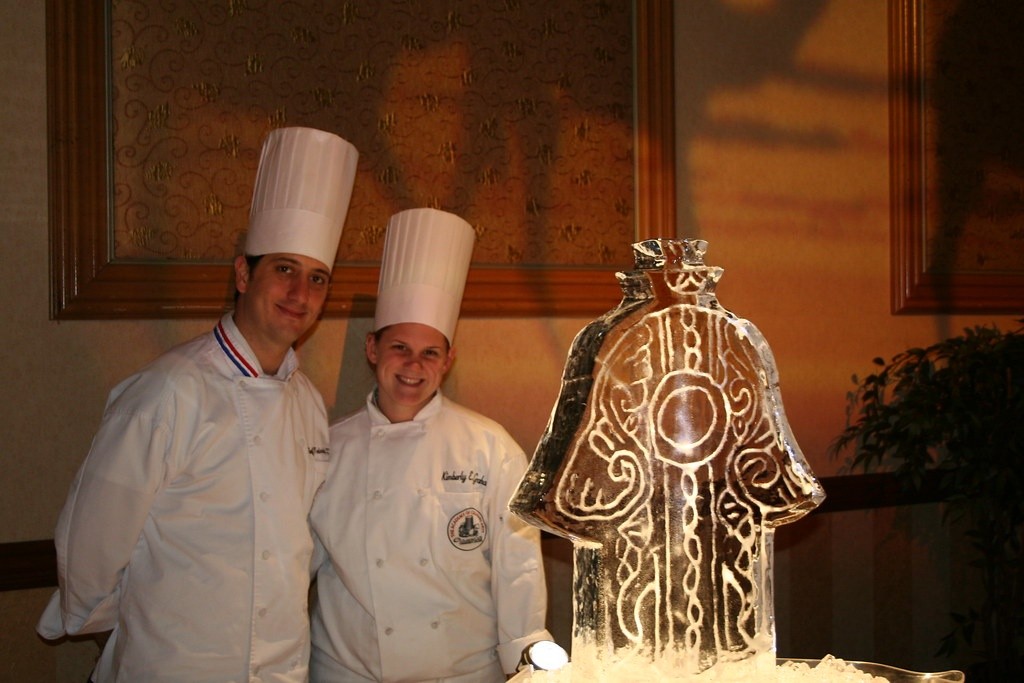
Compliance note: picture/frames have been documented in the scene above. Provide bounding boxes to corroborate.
[46,0,680,321]
[887,0,1024,315]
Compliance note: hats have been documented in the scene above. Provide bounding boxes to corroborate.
[373,207,477,350]
[241,126,362,278]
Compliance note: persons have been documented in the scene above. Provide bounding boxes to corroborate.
[52,209,333,683]
[309,277,558,683]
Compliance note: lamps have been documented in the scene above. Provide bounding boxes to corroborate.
[515,640,569,672]
